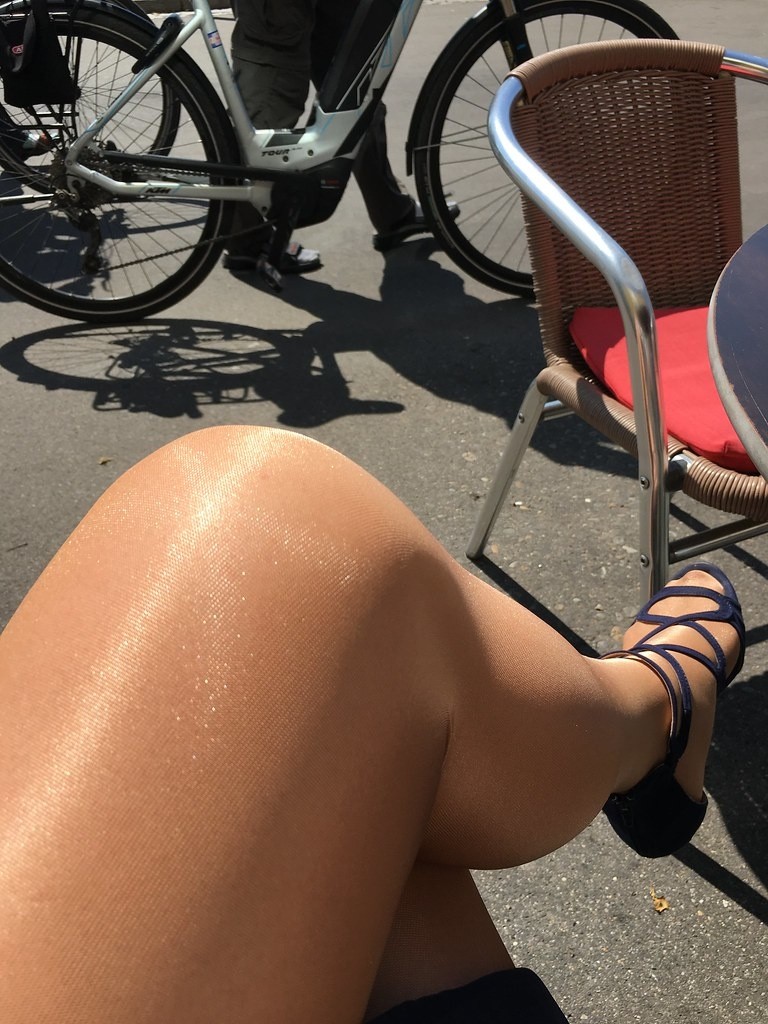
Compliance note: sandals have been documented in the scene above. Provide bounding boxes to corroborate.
[224,242,319,272]
[372,196,461,250]
[602,562,746,859]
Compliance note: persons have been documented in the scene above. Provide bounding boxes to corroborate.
[0,0,459,274]
[0,426,749,1023]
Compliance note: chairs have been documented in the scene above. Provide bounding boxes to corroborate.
[465,39,768,614]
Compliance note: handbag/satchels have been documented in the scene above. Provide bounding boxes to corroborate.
[0,10,82,107]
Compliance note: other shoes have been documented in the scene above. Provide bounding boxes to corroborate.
[21,133,61,167]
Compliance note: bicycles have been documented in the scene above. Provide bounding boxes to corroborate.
[1,1,679,326]
